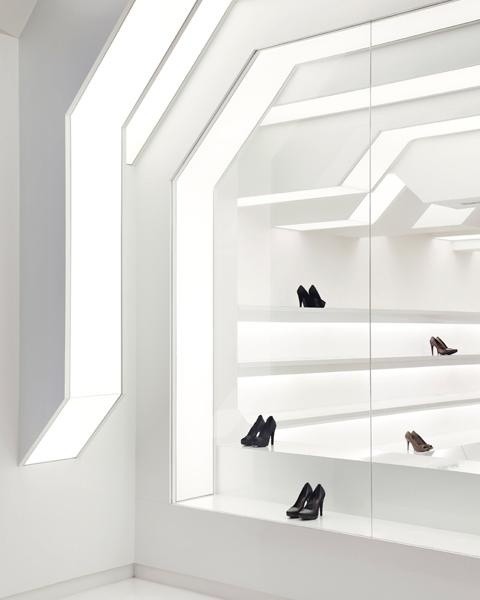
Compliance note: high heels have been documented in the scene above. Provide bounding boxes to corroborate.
[429,336,457,355]
[287,482,326,519]
[241,415,276,448]
[295,284,326,307]
[405,430,433,451]
